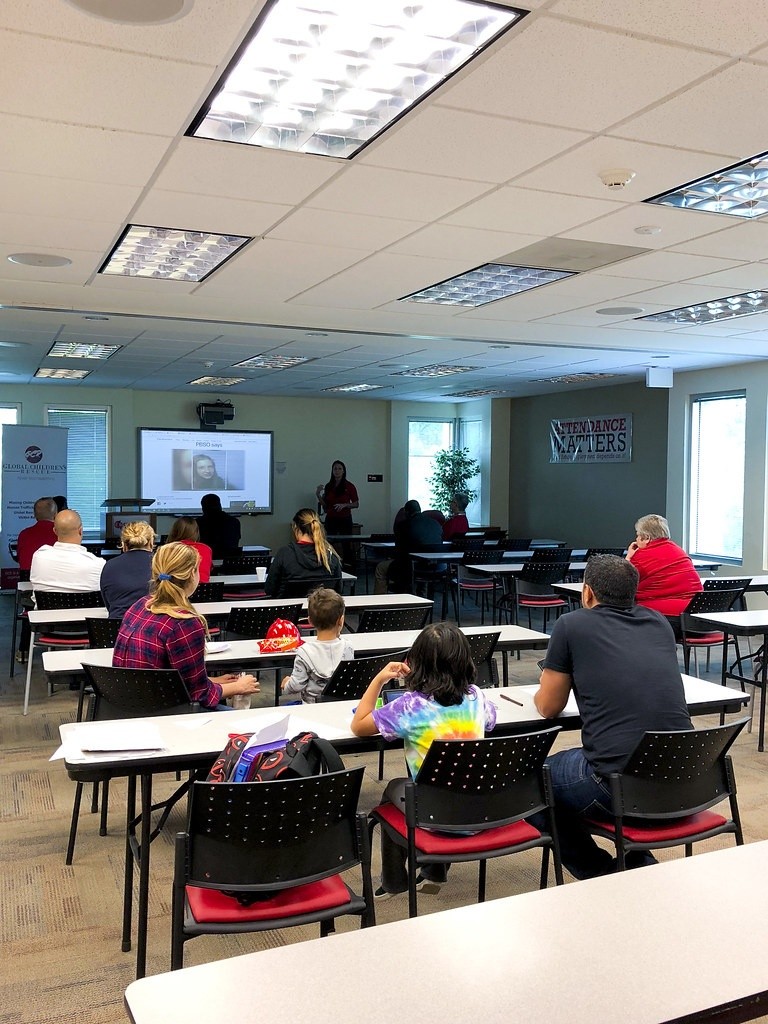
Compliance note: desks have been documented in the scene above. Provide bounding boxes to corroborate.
[326,532,486,571]
[466,558,722,624]
[60,674,753,977]
[100,546,270,556]
[122,840,768,1024]
[11,572,358,678]
[409,549,627,623]
[41,625,557,719]
[689,609,768,751]
[550,576,765,620]
[23,593,437,715]
[9,539,161,556]
[361,539,567,594]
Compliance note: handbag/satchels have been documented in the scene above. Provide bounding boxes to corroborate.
[208,730,346,909]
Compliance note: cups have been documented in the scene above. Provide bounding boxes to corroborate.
[256,567,267,581]
[233,671,256,709]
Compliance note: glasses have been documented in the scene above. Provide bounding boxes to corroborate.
[448,499,454,503]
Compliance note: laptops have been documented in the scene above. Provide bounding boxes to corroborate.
[382,689,407,708]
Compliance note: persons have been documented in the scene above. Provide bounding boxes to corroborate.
[16,509,107,663]
[193,455,225,490]
[280,586,355,705]
[166,515,213,584]
[53,496,69,512]
[100,520,158,620]
[108,541,260,712]
[349,619,496,901]
[263,505,344,597]
[441,492,470,541]
[524,552,694,879]
[373,500,448,594]
[625,513,706,640]
[17,497,58,574]
[317,459,360,536]
[194,494,242,564]
[171,449,236,490]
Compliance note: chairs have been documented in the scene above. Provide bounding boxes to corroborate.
[9,531,768,977]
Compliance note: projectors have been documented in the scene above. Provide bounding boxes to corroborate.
[196,402,236,426]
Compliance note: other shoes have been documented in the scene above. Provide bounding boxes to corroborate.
[69,679,80,690]
[15,650,28,662]
[416,874,441,895]
[84,685,94,692]
[374,867,421,901]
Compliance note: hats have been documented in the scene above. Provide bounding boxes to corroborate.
[455,493,469,511]
[257,618,306,653]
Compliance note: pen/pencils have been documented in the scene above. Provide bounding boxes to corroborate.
[500,693,523,707]
